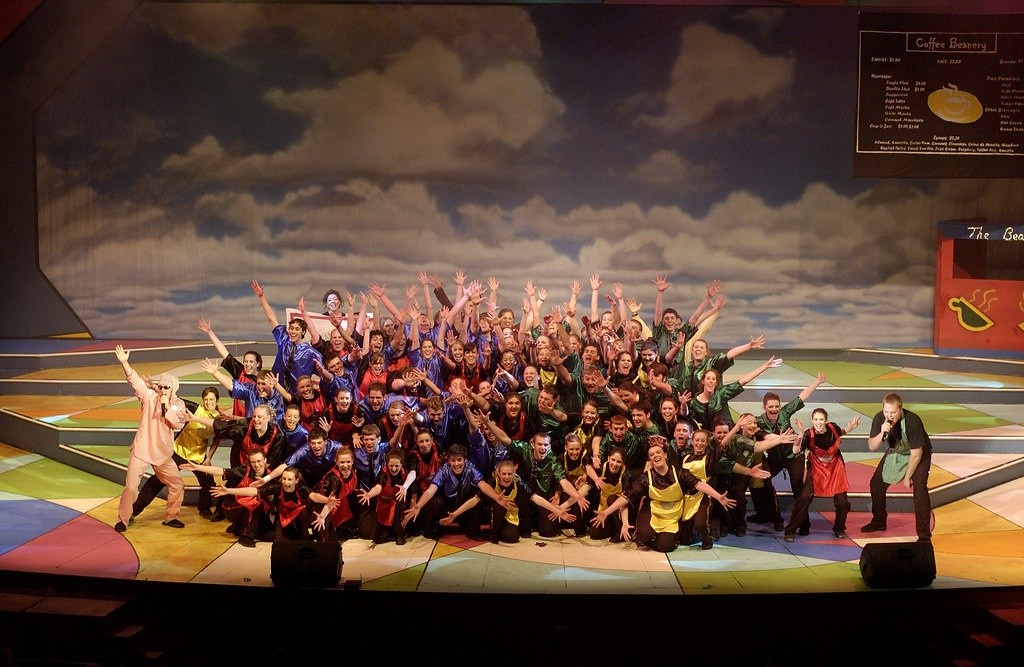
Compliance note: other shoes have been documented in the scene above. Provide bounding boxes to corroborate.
[115,522,126,532]
[785,530,794,542]
[162,519,184,528]
[861,522,886,532]
[832,528,847,538]
[396,536,405,545]
[721,527,729,536]
[701,519,720,550]
[199,509,224,522]
[736,526,746,537]
[774,521,783,532]
[799,527,809,535]
[226,524,256,547]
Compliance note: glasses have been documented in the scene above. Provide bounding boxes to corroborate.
[158,385,170,390]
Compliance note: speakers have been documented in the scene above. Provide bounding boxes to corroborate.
[859,540,937,590]
[270,540,345,588]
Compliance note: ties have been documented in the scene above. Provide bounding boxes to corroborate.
[283,345,296,393]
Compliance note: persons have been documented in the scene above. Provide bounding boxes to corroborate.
[861,392,933,542]
[785,408,862,542]
[114,344,185,533]
[128,269,826,552]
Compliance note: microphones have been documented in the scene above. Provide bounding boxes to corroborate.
[882,420,892,442]
[161,403,165,417]
